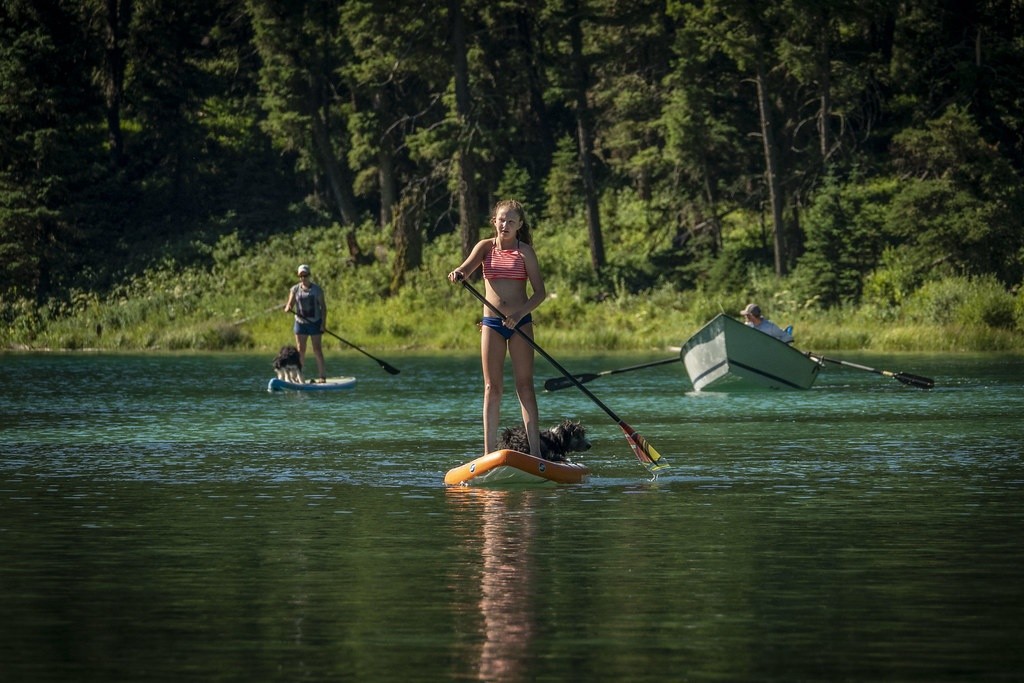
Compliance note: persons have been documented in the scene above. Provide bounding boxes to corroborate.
[740,304,794,346]
[448,199,549,461]
[284,264,327,383]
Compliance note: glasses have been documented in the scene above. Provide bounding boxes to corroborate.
[299,273,310,277]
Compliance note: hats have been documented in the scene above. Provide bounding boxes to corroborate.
[740,304,761,317]
[298,264,310,275]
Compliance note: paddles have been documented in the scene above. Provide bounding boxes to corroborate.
[284,307,401,376]
[456,271,669,475]
[544,357,682,393]
[804,349,935,390]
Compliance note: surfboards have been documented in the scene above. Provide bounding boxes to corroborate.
[444,449,589,487]
[268,377,357,394]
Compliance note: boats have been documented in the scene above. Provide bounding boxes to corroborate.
[681,314,821,392]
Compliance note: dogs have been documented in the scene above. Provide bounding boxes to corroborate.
[495,417,591,463]
[273,344,306,384]
